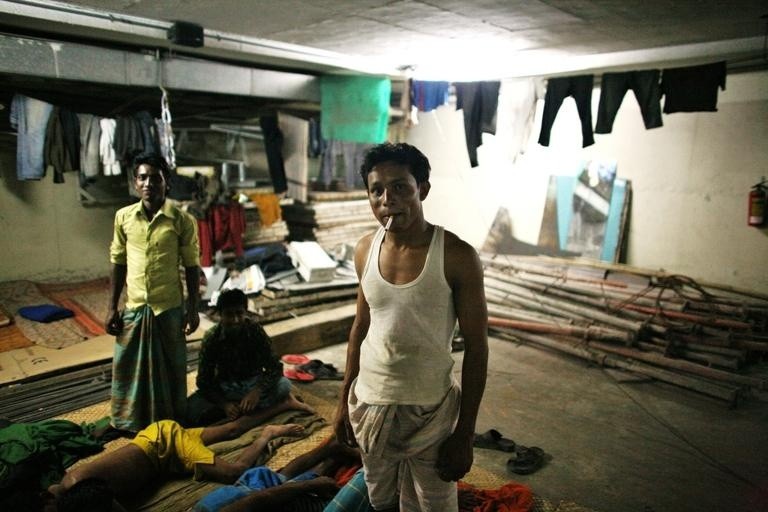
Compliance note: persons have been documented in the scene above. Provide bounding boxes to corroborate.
[334,142,488,512]
[23,390,317,512]
[187,289,293,428]
[105,152,200,435]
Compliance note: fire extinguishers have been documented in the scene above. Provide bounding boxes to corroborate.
[748,180,768,226]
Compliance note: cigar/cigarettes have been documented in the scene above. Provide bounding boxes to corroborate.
[385,215,394,232]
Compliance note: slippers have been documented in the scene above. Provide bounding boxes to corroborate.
[473,430,515,452]
[281,354,344,381]
[507,446,545,475]
